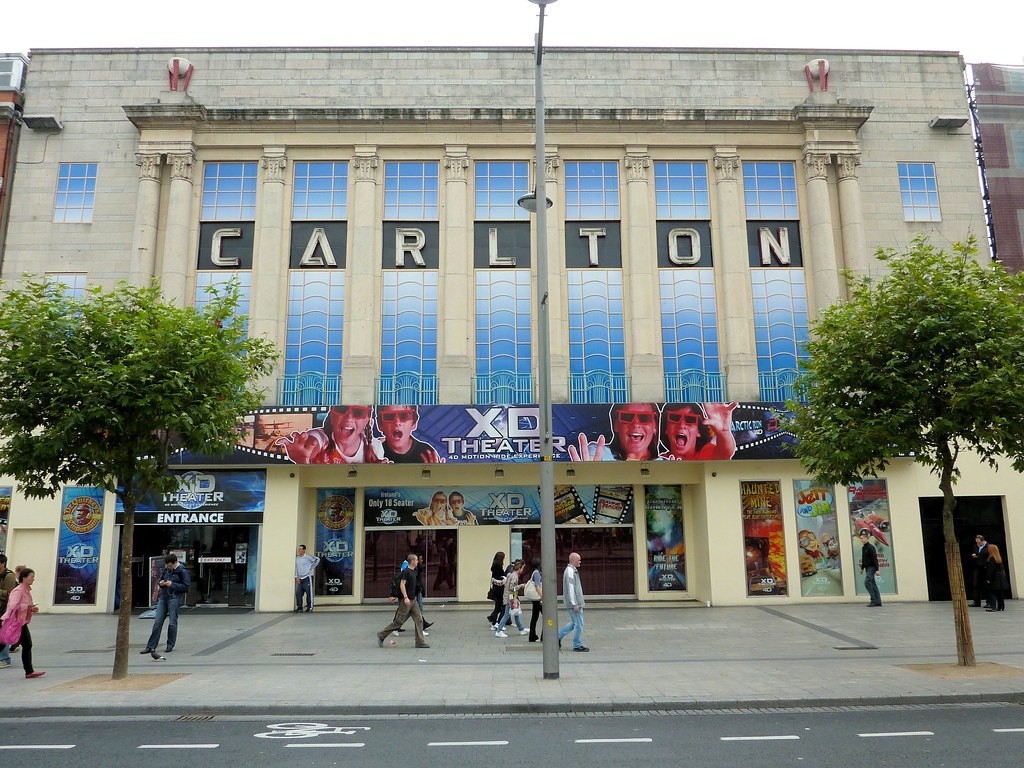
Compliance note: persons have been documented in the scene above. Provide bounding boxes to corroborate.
[568,403,738,461]
[523,529,612,564]
[413,491,479,525]
[0,569,46,678]
[860,530,882,607]
[559,553,589,652]
[967,535,1005,611]
[487,552,530,637]
[0,554,27,668]
[529,557,543,642]
[432,538,455,591]
[141,555,191,654]
[276,405,445,465]
[377,554,435,648]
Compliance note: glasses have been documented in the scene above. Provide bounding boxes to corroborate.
[433,498,446,503]
[665,410,703,425]
[452,500,463,504]
[378,410,414,422]
[613,410,659,425]
[332,406,373,420]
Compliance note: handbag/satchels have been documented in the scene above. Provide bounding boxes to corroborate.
[0,603,23,645]
[508,587,523,615]
[488,588,503,600]
[523,570,543,601]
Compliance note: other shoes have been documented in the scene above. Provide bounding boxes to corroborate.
[415,643,431,648]
[298,607,310,614]
[25,671,45,678]
[165,648,172,652]
[572,646,590,652]
[0,658,11,669]
[140,647,156,654]
[486,616,542,642]
[967,603,1005,612]
[867,603,881,607]
[392,621,435,637]
[377,633,384,648]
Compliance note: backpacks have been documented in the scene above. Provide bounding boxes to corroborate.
[390,572,410,599]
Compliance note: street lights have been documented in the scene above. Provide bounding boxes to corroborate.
[517,0,559,679]
